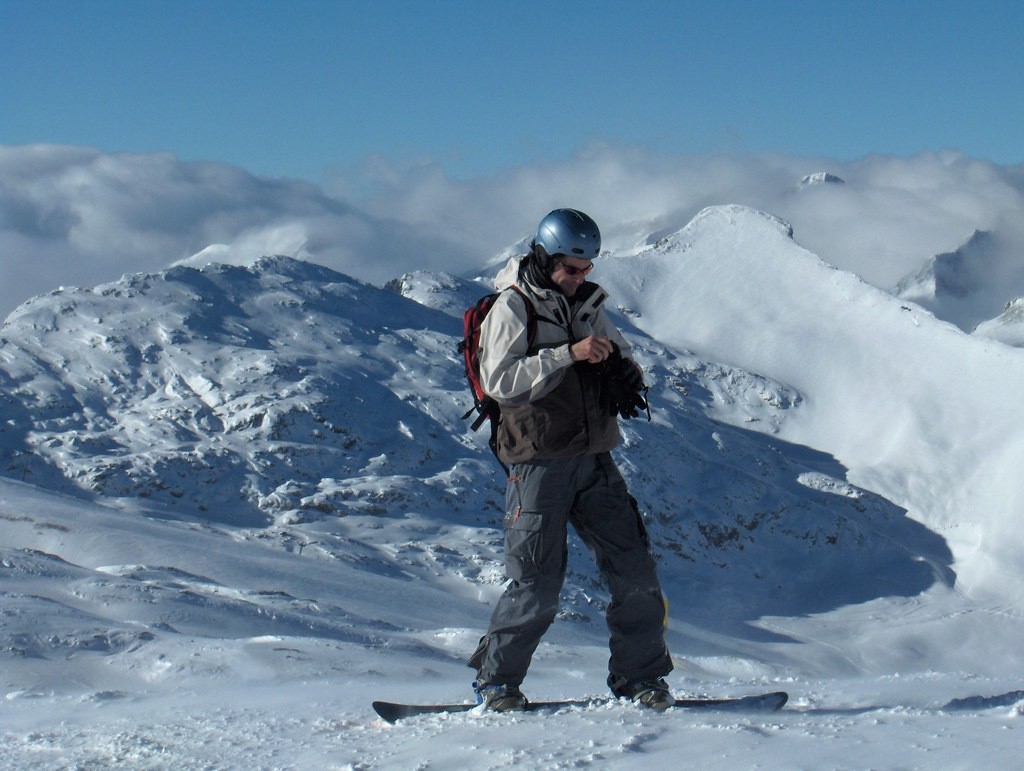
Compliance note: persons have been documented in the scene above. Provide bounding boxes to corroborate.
[467,208,678,712]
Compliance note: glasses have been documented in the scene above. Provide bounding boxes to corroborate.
[559,260,594,276]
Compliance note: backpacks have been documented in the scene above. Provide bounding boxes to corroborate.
[456,285,537,432]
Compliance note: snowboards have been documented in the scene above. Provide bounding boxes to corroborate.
[372,691,787,724]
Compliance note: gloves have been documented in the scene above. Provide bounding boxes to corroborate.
[596,340,647,419]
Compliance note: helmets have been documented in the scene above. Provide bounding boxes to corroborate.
[535,208,601,260]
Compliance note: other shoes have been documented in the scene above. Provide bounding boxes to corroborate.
[613,675,675,712]
[477,676,528,712]
